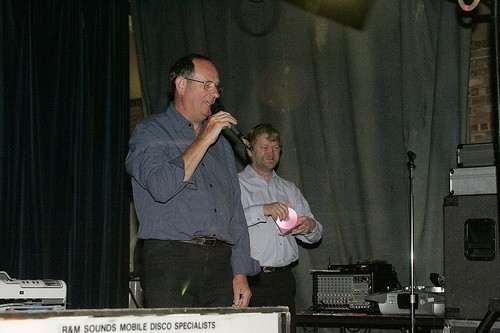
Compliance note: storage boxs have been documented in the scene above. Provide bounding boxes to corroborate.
[449,142,497,195]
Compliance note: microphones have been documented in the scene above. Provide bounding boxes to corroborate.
[210,102,252,150]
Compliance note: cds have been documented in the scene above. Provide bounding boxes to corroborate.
[276,207,298,229]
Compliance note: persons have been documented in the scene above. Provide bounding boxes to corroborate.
[125,55,261,309]
[237,122,323,333]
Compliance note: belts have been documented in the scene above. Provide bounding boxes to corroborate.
[182,237,223,248]
[260,257,300,273]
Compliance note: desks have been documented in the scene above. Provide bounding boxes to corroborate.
[295,308,461,333]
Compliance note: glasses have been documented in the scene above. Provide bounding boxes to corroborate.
[184,77,224,92]
